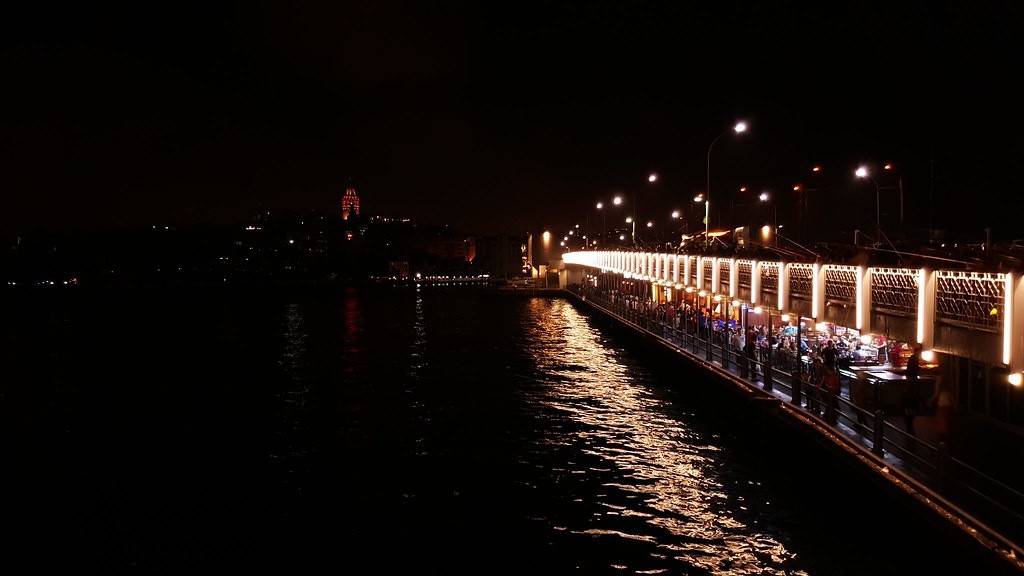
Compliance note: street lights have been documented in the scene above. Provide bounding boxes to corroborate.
[586,203,603,250]
[706,122,745,247]
[857,168,880,242]
[632,175,656,243]
[603,198,622,248]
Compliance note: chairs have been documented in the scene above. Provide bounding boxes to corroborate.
[569,282,909,383]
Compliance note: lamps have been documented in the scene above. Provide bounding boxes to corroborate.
[1007,371,1023,387]
[816,322,826,331]
[922,347,934,362]
[861,335,870,344]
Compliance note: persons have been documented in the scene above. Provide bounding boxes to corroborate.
[611,289,888,427]
